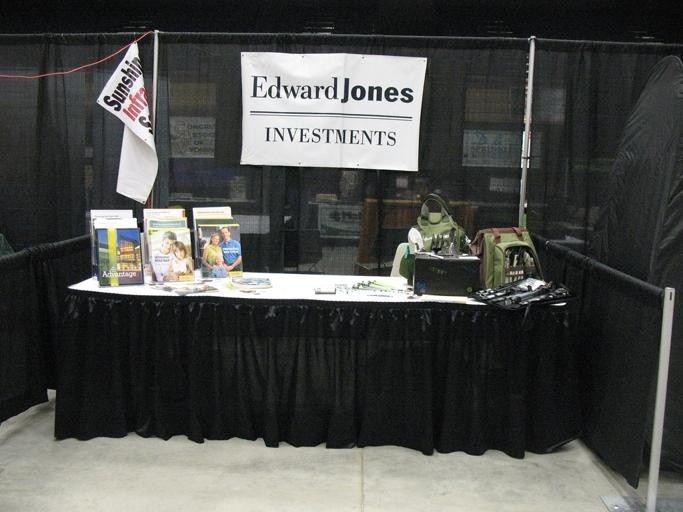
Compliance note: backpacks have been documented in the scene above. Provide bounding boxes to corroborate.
[468,227,544,289]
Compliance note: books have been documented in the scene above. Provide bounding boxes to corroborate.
[88,206,272,296]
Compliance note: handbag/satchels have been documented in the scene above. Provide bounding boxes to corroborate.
[408,194,471,259]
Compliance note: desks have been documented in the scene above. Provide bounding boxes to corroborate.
[54,271,581,459]
